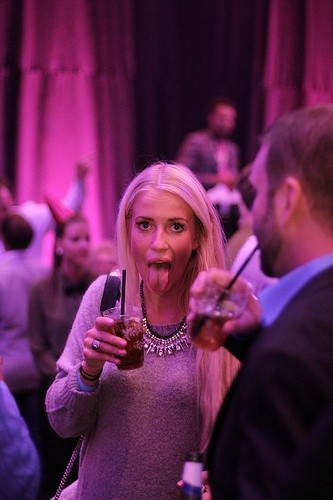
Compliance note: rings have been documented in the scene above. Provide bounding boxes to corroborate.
[92,339,100,350]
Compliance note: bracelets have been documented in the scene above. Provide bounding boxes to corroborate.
[79,361,103,381]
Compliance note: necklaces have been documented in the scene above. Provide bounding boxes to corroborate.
[139,280,191,358]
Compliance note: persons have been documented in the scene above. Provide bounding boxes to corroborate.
[44,165,241,500]
[185,106,333,500]
[0,103,247,500]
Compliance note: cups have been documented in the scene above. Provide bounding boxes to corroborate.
[102,304,144,371]
[186,273,253,352]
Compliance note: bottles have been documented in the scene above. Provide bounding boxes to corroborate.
[178,452,204,500]
